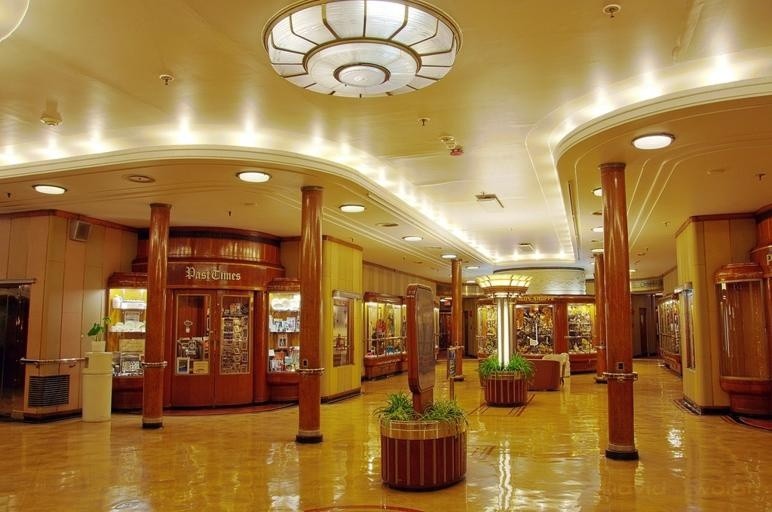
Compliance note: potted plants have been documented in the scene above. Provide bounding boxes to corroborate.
[370,388,470,491]
[87,315,112,351]
[477,353,537,406]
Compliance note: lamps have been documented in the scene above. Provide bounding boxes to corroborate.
[519,243,534,253]
[476,197,503,215]
[262,2,462,100]
